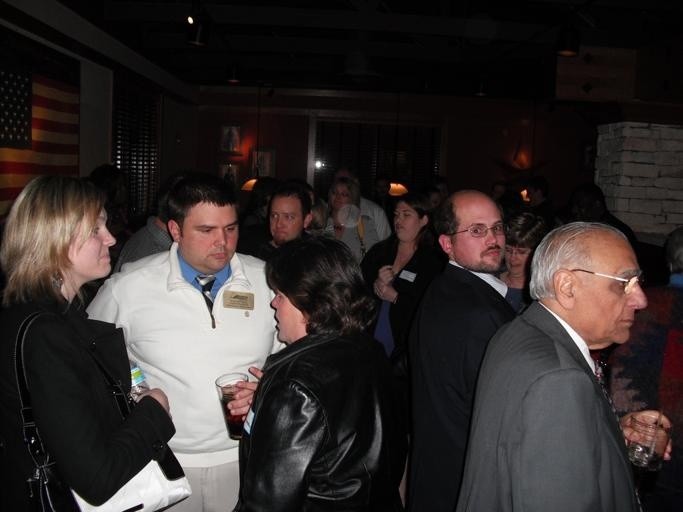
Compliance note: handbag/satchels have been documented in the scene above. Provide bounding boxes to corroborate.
[37,445,192,512]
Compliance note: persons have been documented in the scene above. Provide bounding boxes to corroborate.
[2,173,682,510]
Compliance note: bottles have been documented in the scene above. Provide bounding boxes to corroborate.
[126,364,149,404]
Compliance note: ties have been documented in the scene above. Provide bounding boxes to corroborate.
[596,366,646,512]
[195,275,216,313]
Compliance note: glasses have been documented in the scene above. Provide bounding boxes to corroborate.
[445,223,505,237]
[570,269,643,295]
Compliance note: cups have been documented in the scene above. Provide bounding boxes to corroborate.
[213,371,248,439]
[623,408,674,473]
